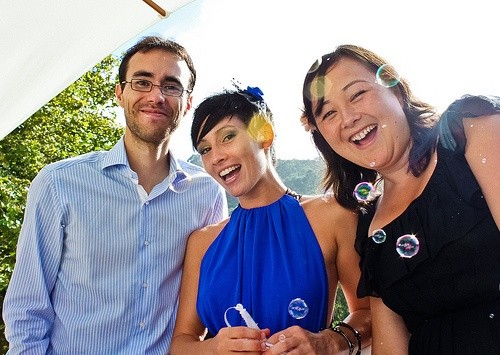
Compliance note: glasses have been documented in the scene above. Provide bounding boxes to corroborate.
[122,79,192,97]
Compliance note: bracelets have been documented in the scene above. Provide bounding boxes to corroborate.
[328,327,355,355]
[336,321,362,355]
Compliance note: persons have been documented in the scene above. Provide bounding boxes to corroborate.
[2,34,229,354]
[299,44,500,355]
[169,85,373,355]
